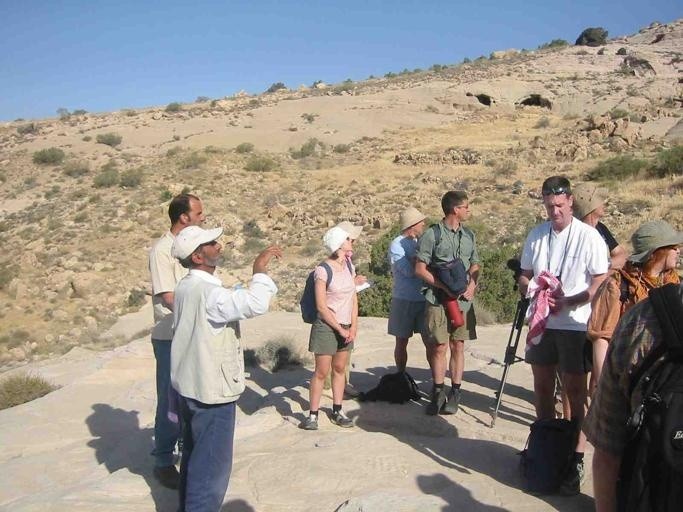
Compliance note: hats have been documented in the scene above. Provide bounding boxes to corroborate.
[572,182,610,220]
[323,226,350,255]
[400,207,428,232]
[625,219,683,267]
[170,223,224,261]
[336,220,363,240]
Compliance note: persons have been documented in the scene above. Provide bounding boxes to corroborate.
[145,194,205,489]
[168,227,284,512]
[303,226,357,430]
[337,221,367,397]
[414,191,482,417]
[387,206,428,377]
[581,283,682,512]
[517,176,682,495]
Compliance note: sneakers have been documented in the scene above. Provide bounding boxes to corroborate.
[298,418,318,429]
[425,388,446,415]
[440,389,461,414]
[560,455,586,496]
[330,409,353,429]
[344,383,361,398]
[153,460,182,490]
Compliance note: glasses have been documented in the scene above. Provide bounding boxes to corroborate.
[541,187,573,197]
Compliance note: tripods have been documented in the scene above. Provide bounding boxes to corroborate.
[490,293,530,427]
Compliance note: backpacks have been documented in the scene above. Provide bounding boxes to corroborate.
[617,282,682,512]
[299,257,353,325]
[517,414,576,495]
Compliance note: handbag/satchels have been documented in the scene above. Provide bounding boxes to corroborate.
[434,256,468,295]
[376,371,419,404]
